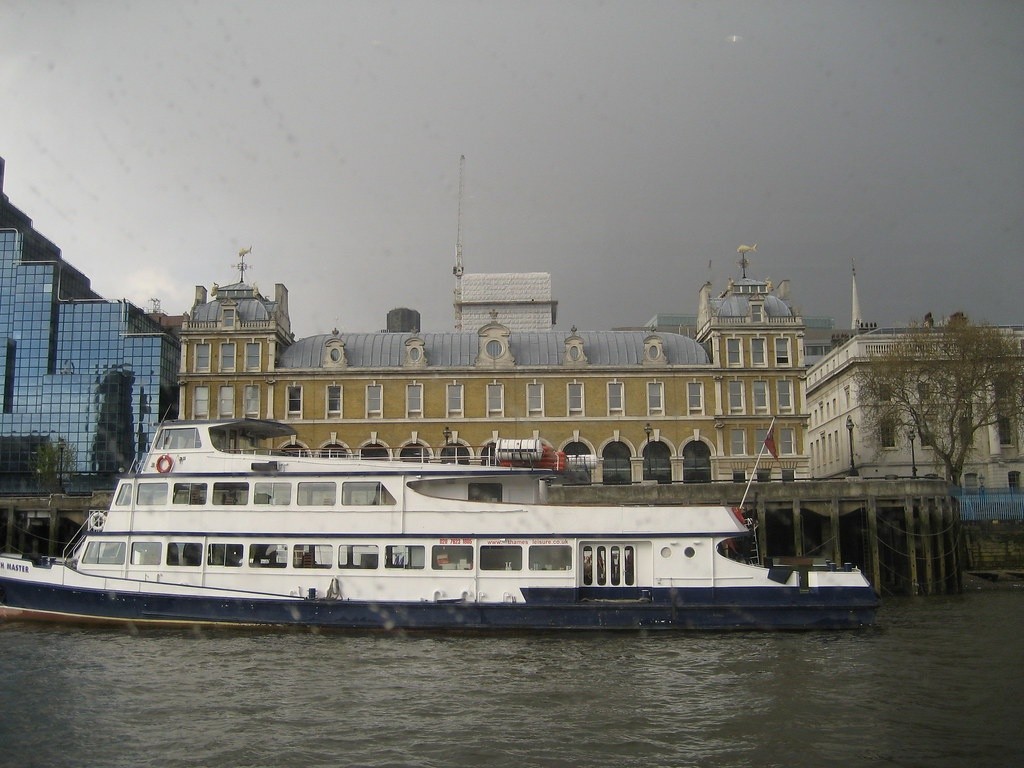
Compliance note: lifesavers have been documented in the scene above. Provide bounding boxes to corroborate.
[156,456,173,474]
[90,512,106,531]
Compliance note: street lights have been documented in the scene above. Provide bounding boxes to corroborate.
[441,427,452,464]
[58,438,67,493]
[645,423,653,483]
[907,429,918,480]
[845,415,858,477]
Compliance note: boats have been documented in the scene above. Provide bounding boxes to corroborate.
[0,404,880,634]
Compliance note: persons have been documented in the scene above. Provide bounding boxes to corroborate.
[584,549,633,582]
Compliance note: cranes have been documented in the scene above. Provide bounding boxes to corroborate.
[452,153,465,324]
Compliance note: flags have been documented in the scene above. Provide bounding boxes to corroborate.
[764,422,778,461]
